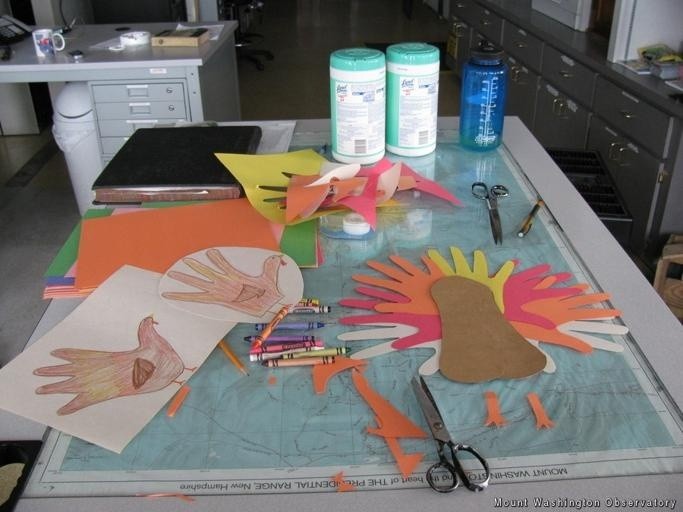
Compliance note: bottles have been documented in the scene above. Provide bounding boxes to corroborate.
[328,46,385,165]
[459,37,509,153]
[386,41,440,158]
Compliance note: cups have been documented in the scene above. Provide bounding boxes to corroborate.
[32,28,64,57]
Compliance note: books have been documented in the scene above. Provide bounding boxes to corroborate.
[618,60,651,75]
[91,126,262,206]
[151,28,209,47]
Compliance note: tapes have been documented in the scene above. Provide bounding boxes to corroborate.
[120,31,151,45]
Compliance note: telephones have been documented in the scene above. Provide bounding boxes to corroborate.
[0,14,32,44]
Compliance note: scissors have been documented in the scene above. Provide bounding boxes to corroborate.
[411,376,492,492]
[472,183,511,245]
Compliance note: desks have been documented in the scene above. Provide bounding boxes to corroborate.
[1,114,683,510]
[0,23,243,169]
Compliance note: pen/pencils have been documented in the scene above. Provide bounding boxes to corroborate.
[518,200,545,238]
[218,299,351,376]
[318,144,327,156]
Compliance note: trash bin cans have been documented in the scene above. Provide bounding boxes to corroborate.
[52,82,109,222]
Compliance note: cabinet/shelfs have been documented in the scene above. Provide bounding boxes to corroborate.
[446,1,475,80]
[502,19,536,130]
[586,64,683,259]
[534,38,597,153]
[475,4,502,48]
